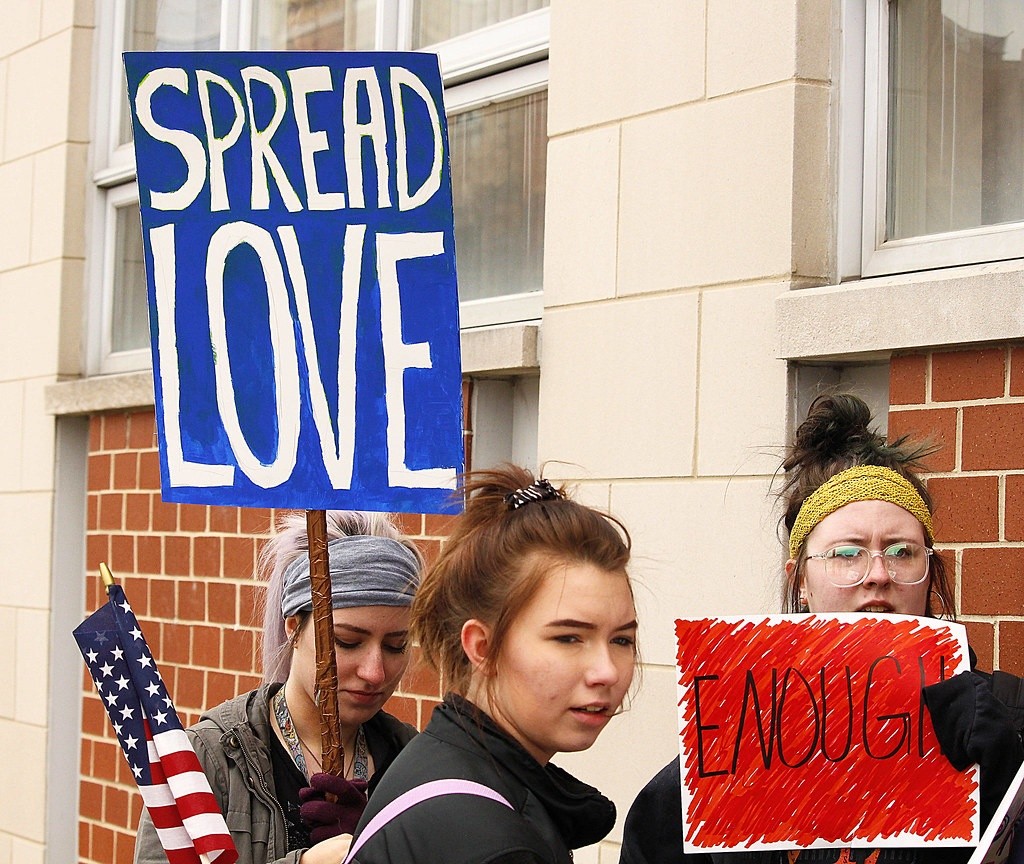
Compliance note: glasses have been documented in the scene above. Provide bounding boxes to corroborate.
[803,542,934,589]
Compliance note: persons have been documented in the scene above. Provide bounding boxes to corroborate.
[338,463,639,864]
[132,506,424,863]
[619,393,1023,864]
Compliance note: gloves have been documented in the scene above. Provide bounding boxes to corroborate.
[298,772,369,844]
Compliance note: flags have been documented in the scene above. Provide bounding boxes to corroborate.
[71,585,239,864]
